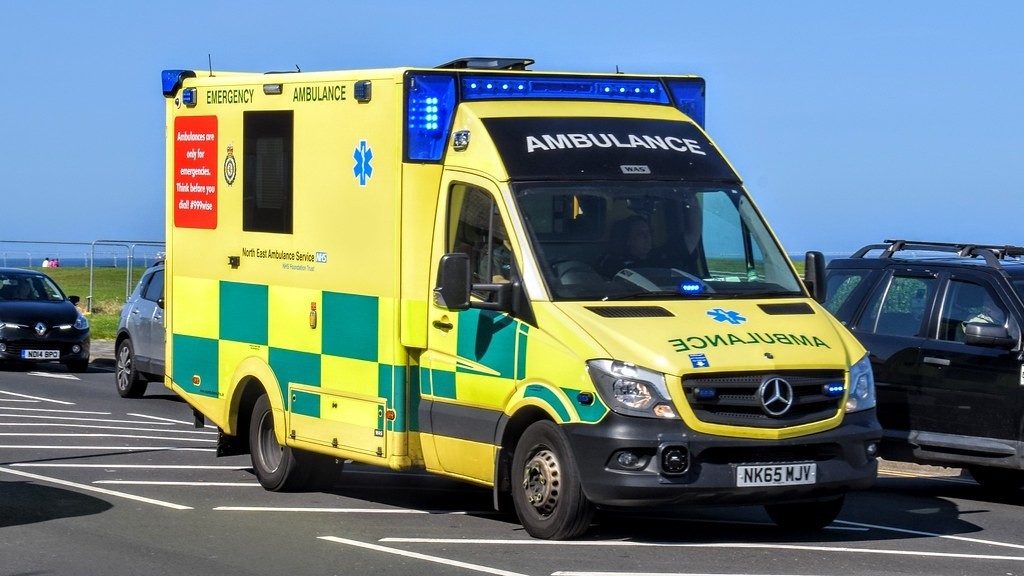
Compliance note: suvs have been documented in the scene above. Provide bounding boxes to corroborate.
[113,257,166,398]
[817,237,1024,491]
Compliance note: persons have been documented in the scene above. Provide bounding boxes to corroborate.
[15,281,38,300]
[954,285,1021,345]
[42,258,60,268]
[478,213,511,302]
[600,184,703,293]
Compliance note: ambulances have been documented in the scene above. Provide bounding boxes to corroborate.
[158,55,885,543]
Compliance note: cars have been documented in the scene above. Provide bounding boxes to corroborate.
[0,267,90,372]
